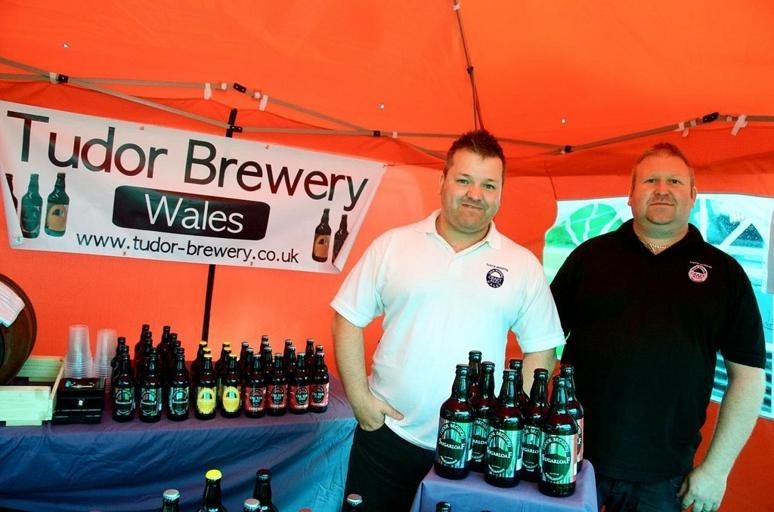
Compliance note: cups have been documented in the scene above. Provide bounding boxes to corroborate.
[94,328,117,398]
[63,324,93,377]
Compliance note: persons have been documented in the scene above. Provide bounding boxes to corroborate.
[550,142,766,512]
[328,130,568,512]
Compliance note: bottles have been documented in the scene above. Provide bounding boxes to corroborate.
[262,334,269,341]
[304,338,314,374]
[221,353,242,418]
[436,502,452,512]
[315,345,324,359]
[165,354,190,421]
[162,489,181,512]
[537,374,577,497]
[20,174,43,238]
[161,332,177,363]
[190,340,207,385]
[195,470,229,512]
[6,173,18,214]
[471,361,497,472]
[244,354,267,418]
[111,354,135,423]
[345,493,365,512]
[164,340,180,379]
[282,339,293,368]
[45,172,70,237]
[242,348,253,385]
[217,346,231,399]
[157,326,171,353]
[560,366,584,474]
[136,331,152,369]
[254,469,279,512]
[242,498,262,512]
[261,347,273,383]
[193,347,212,386]
[311,352,330,413]
[236,342,249,372]
[509,359,531,420]
[484,368,524,487]
[111,337,126,369]
[285,345,296,383]
[312,209,332,262]
[259,341,268,362]
[139,349,162,423]
[134,324,149,367]
[332,215,350,263]
[267,353,288,417]
[522,368,549,483]
[109,345,130,403]
[435,364,472,480]
[193,352,218,421]
[468,350,482,406]
[213,342,230,379]
[138,340,152,375]
[290,352,310,414]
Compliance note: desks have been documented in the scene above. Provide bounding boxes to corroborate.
[414,458,604,511]
[0,363,357,509]
